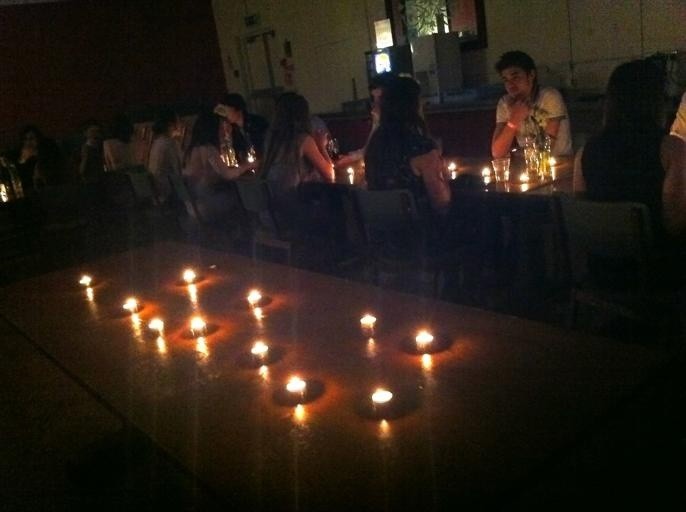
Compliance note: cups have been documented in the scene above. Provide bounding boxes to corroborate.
[328,138,339,161]
[492,158,511,182]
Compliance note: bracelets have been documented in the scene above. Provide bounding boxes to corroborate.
[505,121,516,130]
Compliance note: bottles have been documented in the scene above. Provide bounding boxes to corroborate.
[520,136,540,182]
[540,134,560,183]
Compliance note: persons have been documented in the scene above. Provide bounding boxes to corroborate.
[487,50,572,164]
[570,58,686,245]
[13,90,337,227]
[338,72,452,223]
